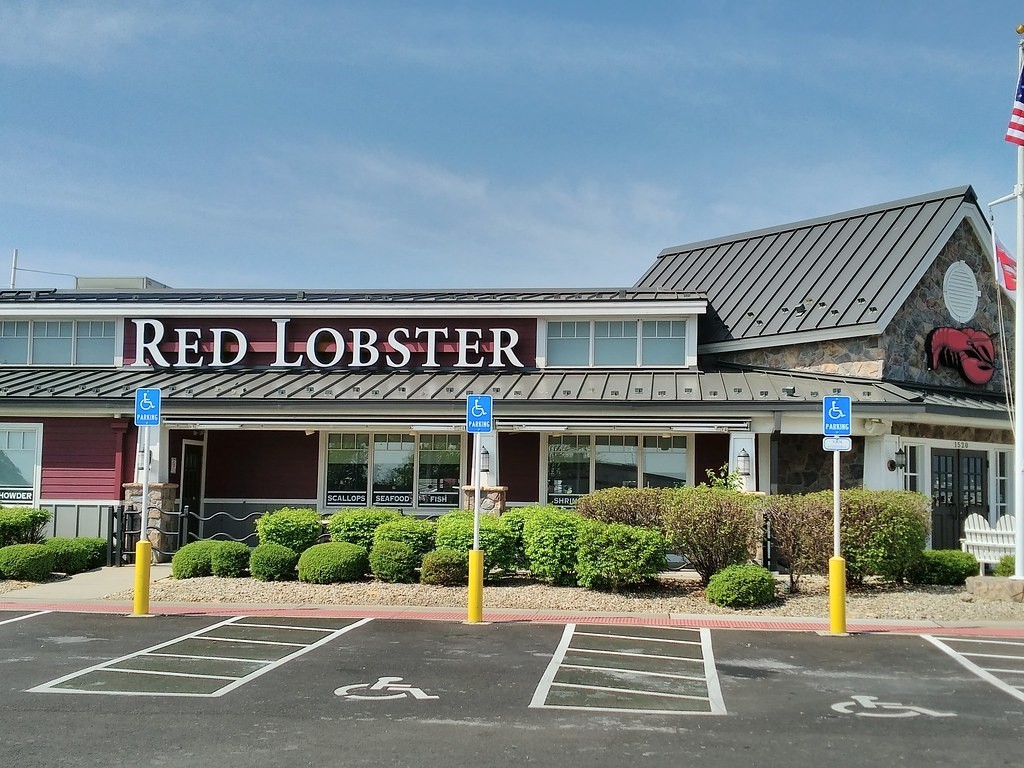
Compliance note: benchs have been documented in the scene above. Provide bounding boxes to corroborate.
[959,513,1016,577]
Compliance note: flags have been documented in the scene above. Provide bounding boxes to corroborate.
[1004,57,1024,147]
[993,231,1016,291]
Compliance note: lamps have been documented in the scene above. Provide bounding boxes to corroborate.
[480,446,489,472]
[137,444,151,471]
[736,448,752,479]
[888,448,906,472]
